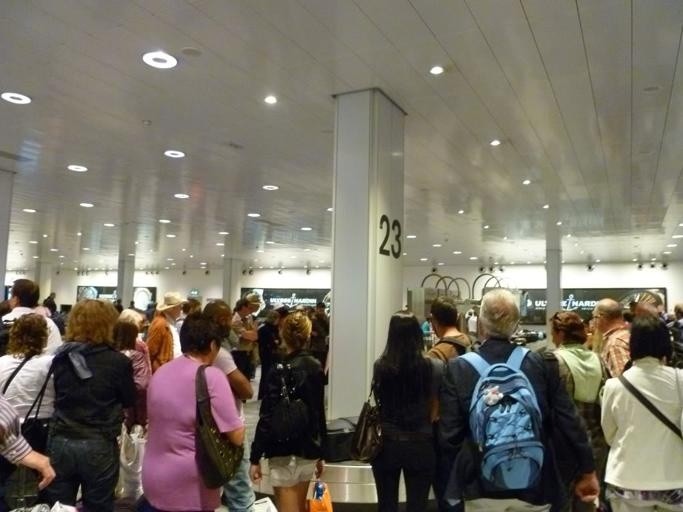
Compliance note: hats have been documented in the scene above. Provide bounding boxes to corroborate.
[247,293,262,304]
[156,292,189,311]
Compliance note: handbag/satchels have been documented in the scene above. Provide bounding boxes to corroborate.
[270,360,308,444]
[21,417,46,452]
[352,373,383,463]
[116,423,146,500]
[196,365,243,488]
[308,478,333,512]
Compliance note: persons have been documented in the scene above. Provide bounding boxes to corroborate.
[425,296,472,505]
[438,287,598,511]
[38,300,133,512]
[1,313,58,506]
[421,298,683,459]
[0,395,56,492]
[600,316,683,512]
[250,313,325,512]
[133,313,246,510]
[372,310,443,511]
[1,278,331,458]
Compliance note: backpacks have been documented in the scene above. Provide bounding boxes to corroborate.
[457,346,545,492]
